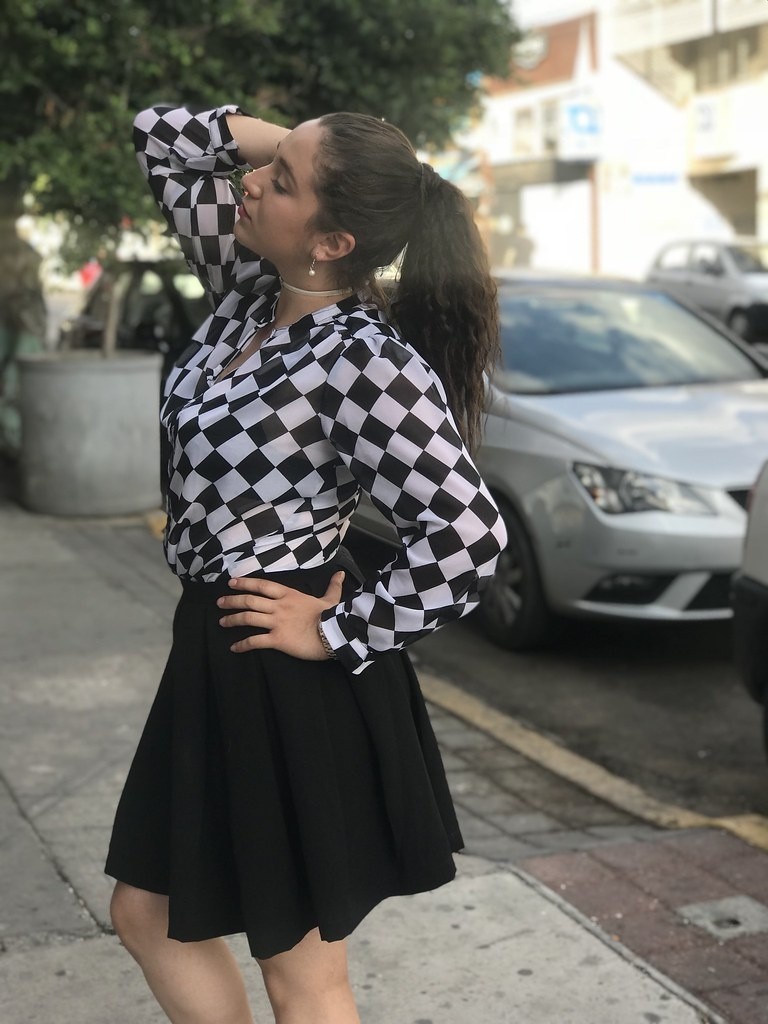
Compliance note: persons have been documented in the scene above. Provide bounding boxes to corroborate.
[101,106,506,1024]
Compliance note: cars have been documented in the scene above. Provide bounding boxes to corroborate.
[71,258,218,364]
[369,267,768,650]
[651,242,768,345]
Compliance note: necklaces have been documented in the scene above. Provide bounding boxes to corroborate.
[283,280,353,296]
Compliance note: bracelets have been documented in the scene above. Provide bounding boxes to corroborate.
[317,621,339,659]
